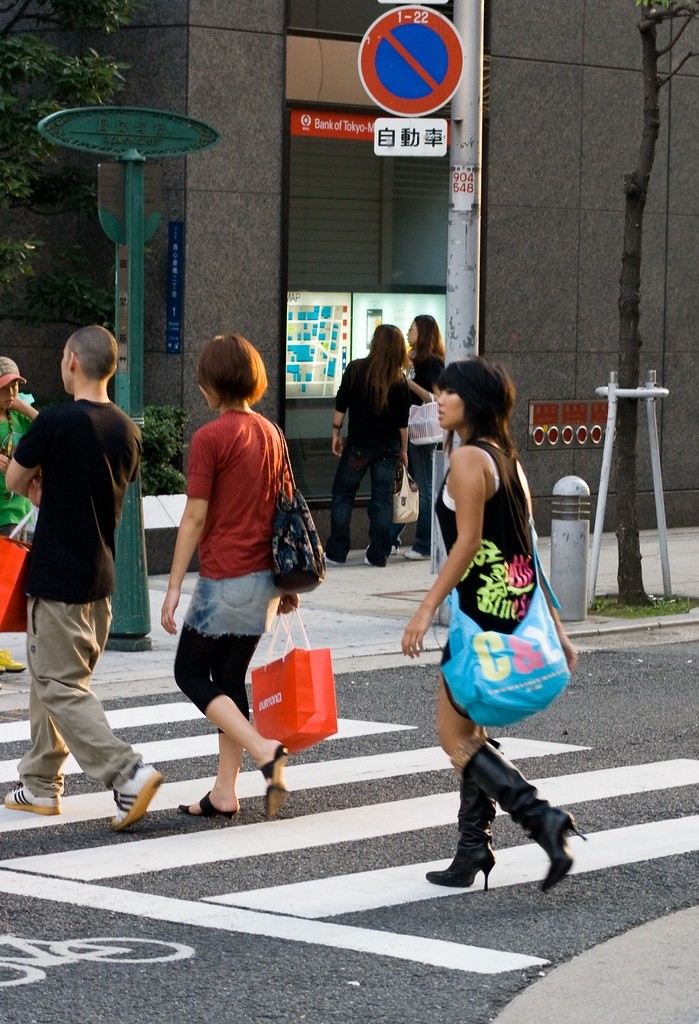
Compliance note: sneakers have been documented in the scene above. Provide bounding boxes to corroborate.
[112,764,163,830]
[5,782,61,814]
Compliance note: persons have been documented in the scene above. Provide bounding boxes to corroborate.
[324,314,446,567]
[0,356,39,672]
[403,359,587,894]
[5,325,164,831]
[161,334,299,819]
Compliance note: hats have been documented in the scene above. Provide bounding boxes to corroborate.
[0,357,26,388]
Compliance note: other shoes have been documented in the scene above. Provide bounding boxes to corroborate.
[403,550,431,559]
[324,552,338,563]
[364,557,371,565]
[390,546,399,554]
[0,650,26,672]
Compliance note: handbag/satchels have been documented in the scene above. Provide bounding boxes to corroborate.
[408,392,443,444]
[271,422,327,592]
[440,586,571,725]
[251,607,338,752]
[392,463,419,523]
[0,506,37,632]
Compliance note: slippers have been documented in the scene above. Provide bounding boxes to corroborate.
[260,746,288,820]
[179,791,236,820]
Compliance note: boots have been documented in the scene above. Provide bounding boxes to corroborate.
[426,738,504,891]
[463,741,587,894]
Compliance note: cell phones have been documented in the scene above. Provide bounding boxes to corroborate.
[367,310,383,348]
